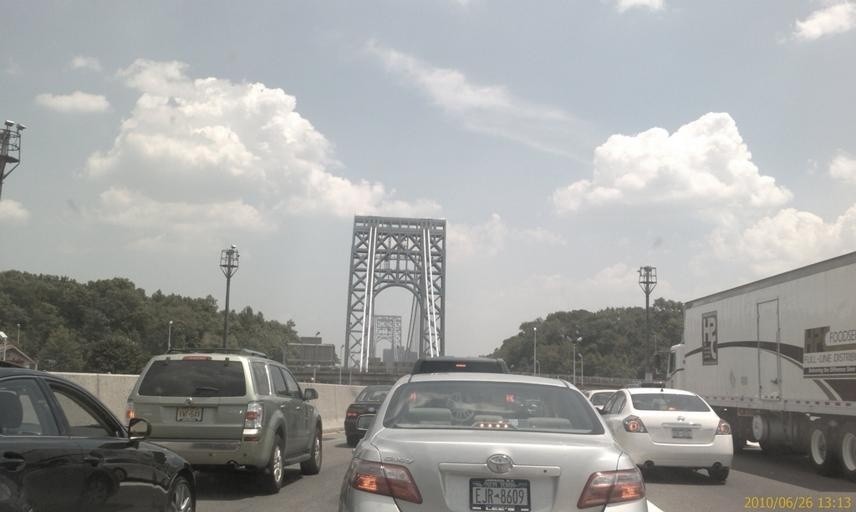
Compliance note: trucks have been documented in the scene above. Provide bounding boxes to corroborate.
[666,249,856,484]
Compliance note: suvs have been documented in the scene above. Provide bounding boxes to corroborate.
[408,355,516,416]
[126,352,323,495]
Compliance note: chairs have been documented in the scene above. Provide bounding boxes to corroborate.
[407,408,451,425]
[528,417,572,430]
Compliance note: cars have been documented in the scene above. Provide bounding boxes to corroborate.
[0,359,197,511]
[580,387,624,419]
[337,373,649,512]
[598,388,733,483]
[343,384,423,448]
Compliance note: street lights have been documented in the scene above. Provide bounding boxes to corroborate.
[312,331,320,383]
[532,326,541,376]
[220,244,239,349]
[167,320,173,351]
[566,336,583,385]
[0,120,28,195]
[338,344,344,384]
[638,265,658,382]
[16,323,20,344]
